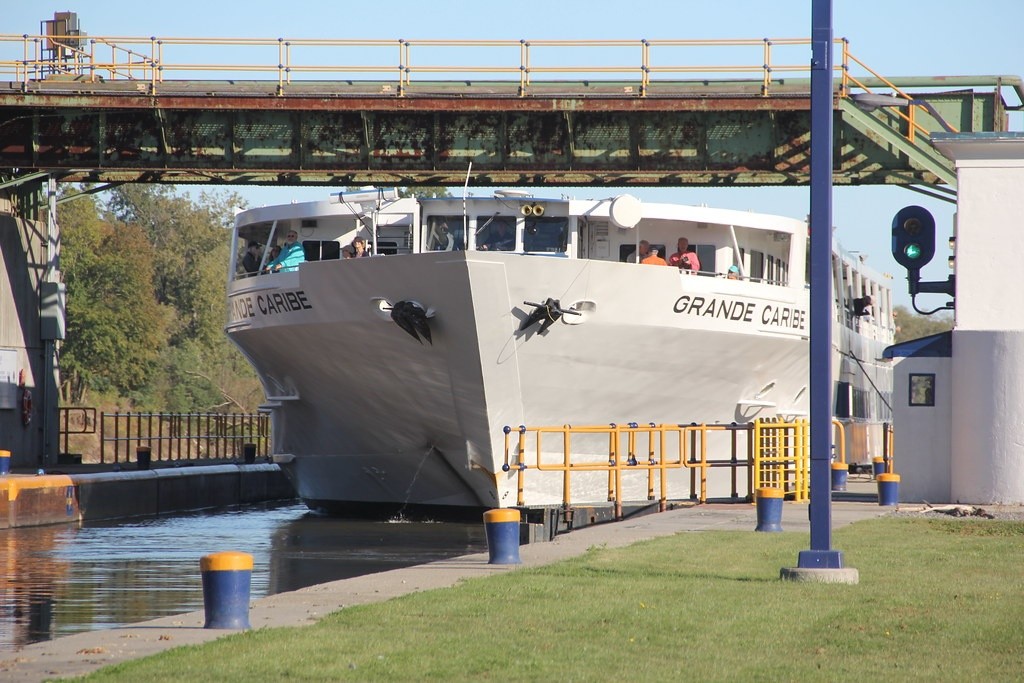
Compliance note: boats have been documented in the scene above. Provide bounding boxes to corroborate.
[223,190,898,525]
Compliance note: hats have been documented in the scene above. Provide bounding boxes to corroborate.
[729,265,737,272]
[339,245,355,255]
[351,237,364,246]
[248,241,262,248]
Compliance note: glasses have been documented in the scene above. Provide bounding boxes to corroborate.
[287,235,295,237]
[252,247,260,249]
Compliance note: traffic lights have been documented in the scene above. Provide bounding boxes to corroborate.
[892,206,935,269]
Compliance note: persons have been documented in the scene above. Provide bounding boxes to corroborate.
[243,230,305,277]
[728,266,738,280]
[343,236,368,258]
[852,296,871,316]
[627,237,700,275]
[435,222,461,251]
[482,221,515,251]
